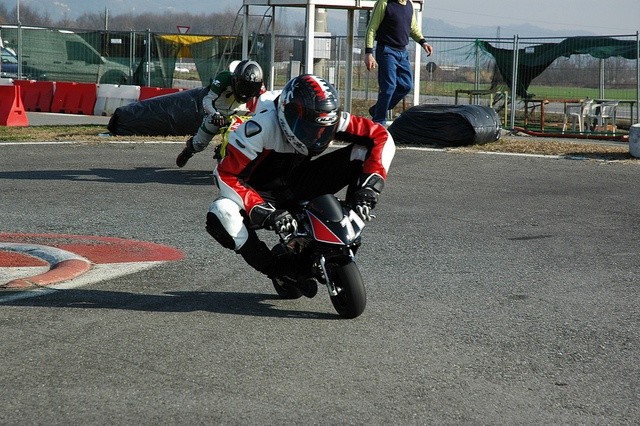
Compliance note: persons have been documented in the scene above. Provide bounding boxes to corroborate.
[365,0,432,127]
[176,59,268,168]
[205,74,396,292]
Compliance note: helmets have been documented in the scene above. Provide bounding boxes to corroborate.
[231,60,264,104]
[277,74,342,158]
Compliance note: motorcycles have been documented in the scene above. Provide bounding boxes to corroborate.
[209,114,257,166]
[269,193,377,318]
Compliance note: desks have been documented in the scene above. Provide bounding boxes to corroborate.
[524,99,637,135]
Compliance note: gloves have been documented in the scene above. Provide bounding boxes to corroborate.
[250,201,299,243]
[345,172,386,222]
[211,111,225,127]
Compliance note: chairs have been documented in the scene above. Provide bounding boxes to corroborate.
[562,98,619,137]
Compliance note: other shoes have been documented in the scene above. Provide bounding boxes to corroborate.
[368,106,376,116]
[270,266,318,299]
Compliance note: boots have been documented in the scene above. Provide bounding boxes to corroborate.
[176,137,199,167]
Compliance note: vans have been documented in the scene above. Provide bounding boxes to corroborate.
[1,25,136,84]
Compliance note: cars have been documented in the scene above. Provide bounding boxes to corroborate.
[173,66,190,73]
[1,47,51,82]
